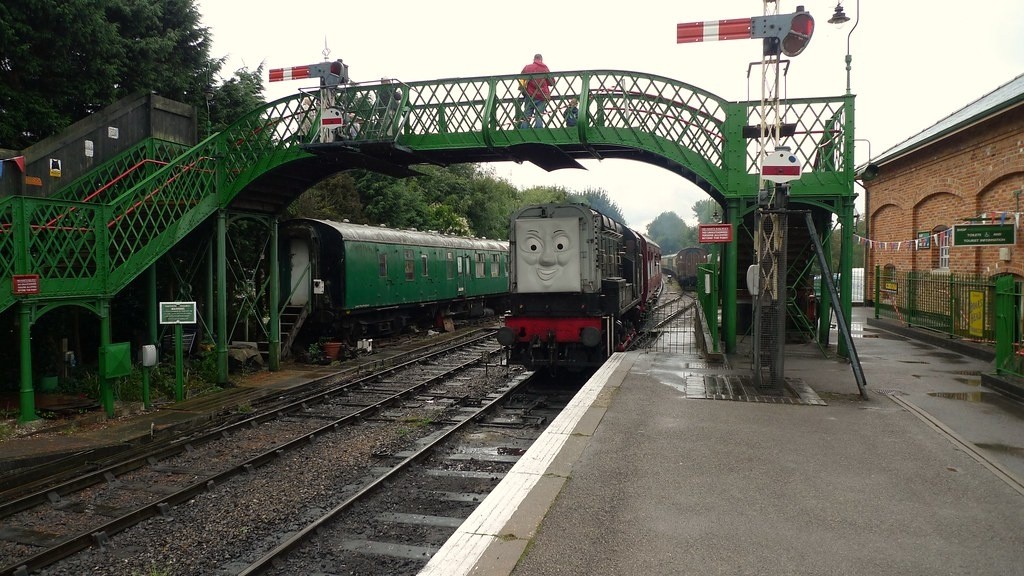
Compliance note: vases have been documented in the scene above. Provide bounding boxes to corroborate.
[322,342,343,361]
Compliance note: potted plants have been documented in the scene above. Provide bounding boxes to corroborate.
[308,343,332,366]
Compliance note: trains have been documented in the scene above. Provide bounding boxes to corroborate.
[497,201,662,375]
[660,246,710,286]
[255,222,511,353]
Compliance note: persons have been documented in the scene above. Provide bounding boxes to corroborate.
[520,54,556,128]
[374,77,404,136]
[563,99,578,126]
